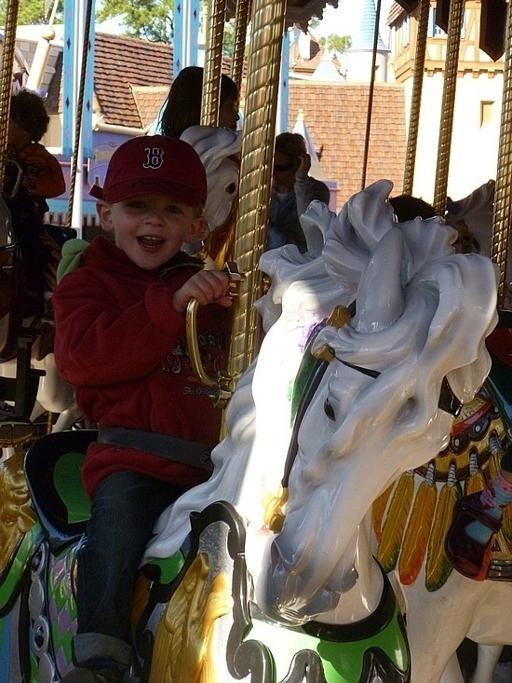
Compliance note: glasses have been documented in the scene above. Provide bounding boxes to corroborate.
[273,165,292,173]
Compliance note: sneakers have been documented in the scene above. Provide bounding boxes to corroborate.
[60,667,106,683]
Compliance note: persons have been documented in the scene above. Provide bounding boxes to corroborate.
[51,136,232,683]
[390,197,437,223]
[160,64,239,135]
[265,130,330,260]
[7,91,65,298]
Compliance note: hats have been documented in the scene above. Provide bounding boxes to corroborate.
[89,133,208,207]
